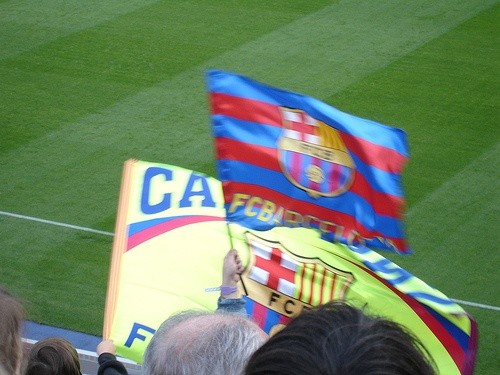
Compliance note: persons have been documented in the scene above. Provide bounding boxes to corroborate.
[0,291,25,375]
[142,309,271,375]
[240,297,440,375]
[26,337,129,375]
[214,248,248,319]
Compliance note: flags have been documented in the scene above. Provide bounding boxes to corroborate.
[205,69,414,255]
[103,159,479,375]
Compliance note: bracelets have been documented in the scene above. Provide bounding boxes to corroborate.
[221,286,237,295]
